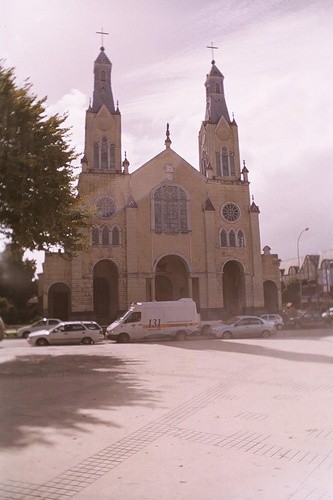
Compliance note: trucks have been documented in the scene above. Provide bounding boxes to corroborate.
[105,298,199,343]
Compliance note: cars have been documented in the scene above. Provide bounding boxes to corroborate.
[15,318,63,338]
[194,306,333,336]
[27,319,104,346]
[208,314,277,339]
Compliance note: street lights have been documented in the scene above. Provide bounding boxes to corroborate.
[297,227,310,310]
